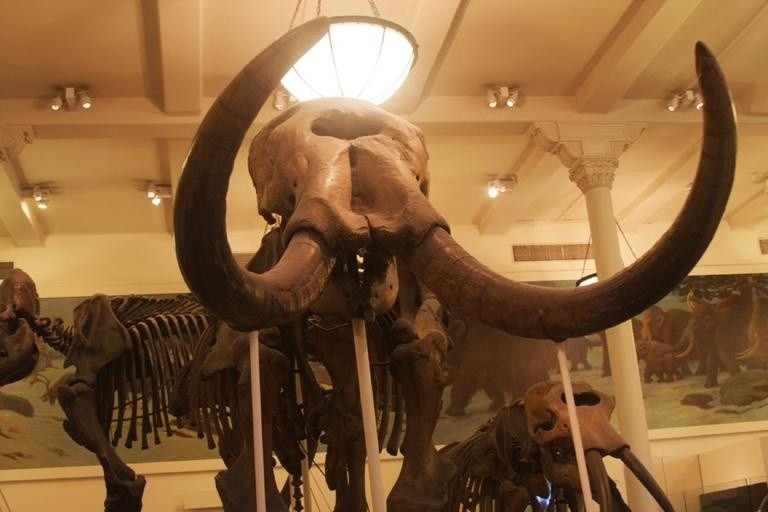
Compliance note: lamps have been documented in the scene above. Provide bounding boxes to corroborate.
[144,181,171,207]
[488,85,520,109]
[22,184,53,211]
[280,1,419,106]
[664,88,705,112]
[49,82,95,113]
